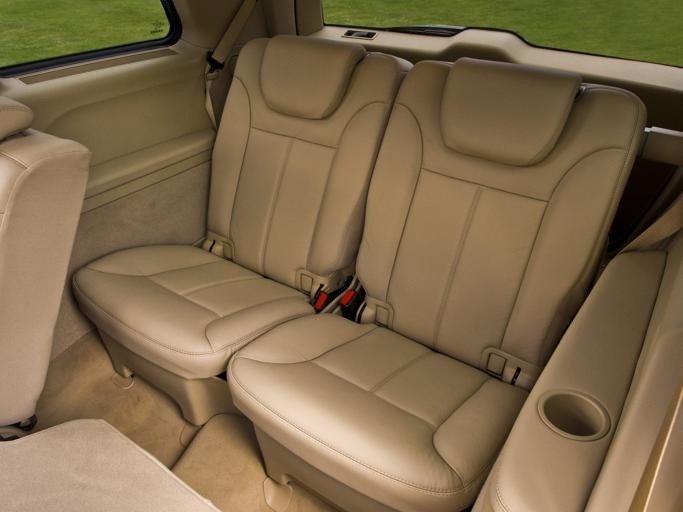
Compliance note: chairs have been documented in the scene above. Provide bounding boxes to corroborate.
[225,54,645,512]
[72,39,416,427]
[0,95,94,432]
